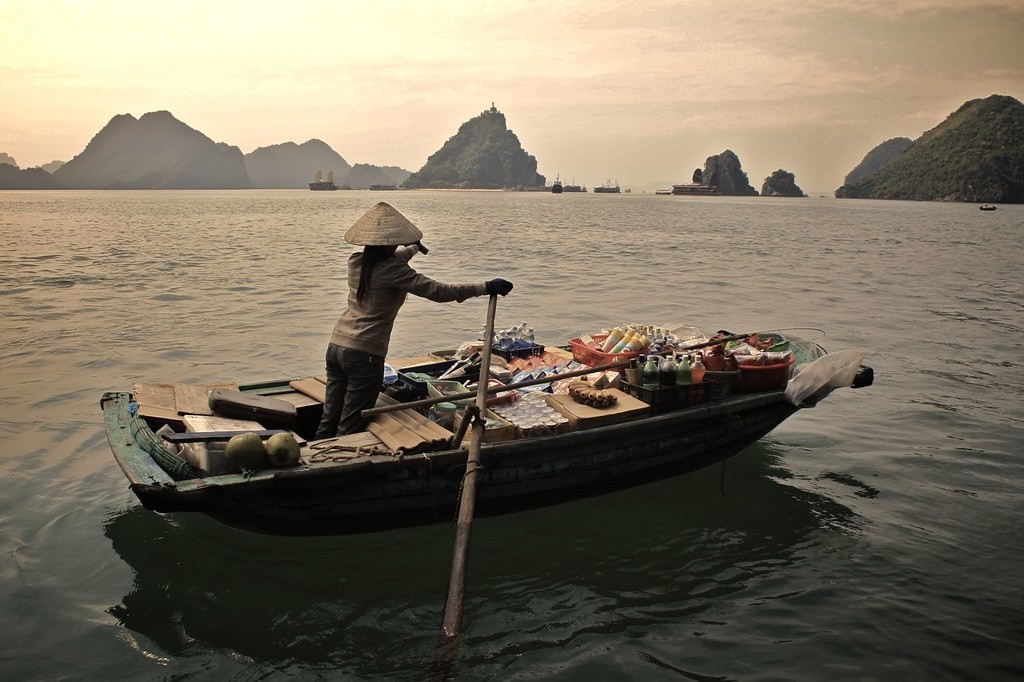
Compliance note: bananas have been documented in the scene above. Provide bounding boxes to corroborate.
[568,382,618,409]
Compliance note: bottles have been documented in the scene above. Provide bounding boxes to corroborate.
[481,321,536,360]
[572,323,708,410]
[425,350,482,380]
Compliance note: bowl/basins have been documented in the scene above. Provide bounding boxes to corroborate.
[734,354,796,392]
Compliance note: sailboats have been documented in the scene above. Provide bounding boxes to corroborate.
[512,174,621,194]
[671,168,720,195]
[308,170,337,191]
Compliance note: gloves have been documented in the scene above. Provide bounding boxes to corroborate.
[485,277,515,297]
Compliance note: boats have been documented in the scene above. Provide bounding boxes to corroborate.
[979,206,997,210]
[98,327,874,540]
[369,184,397,190]
[655,190,672,195]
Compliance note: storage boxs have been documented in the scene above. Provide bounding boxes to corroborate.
[572,331,797,408]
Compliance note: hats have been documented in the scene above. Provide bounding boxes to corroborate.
[344,201,424,246]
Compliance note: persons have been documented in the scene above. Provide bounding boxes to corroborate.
[315,202,515,443]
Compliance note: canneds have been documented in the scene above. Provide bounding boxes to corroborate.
[491,398,570,440]
